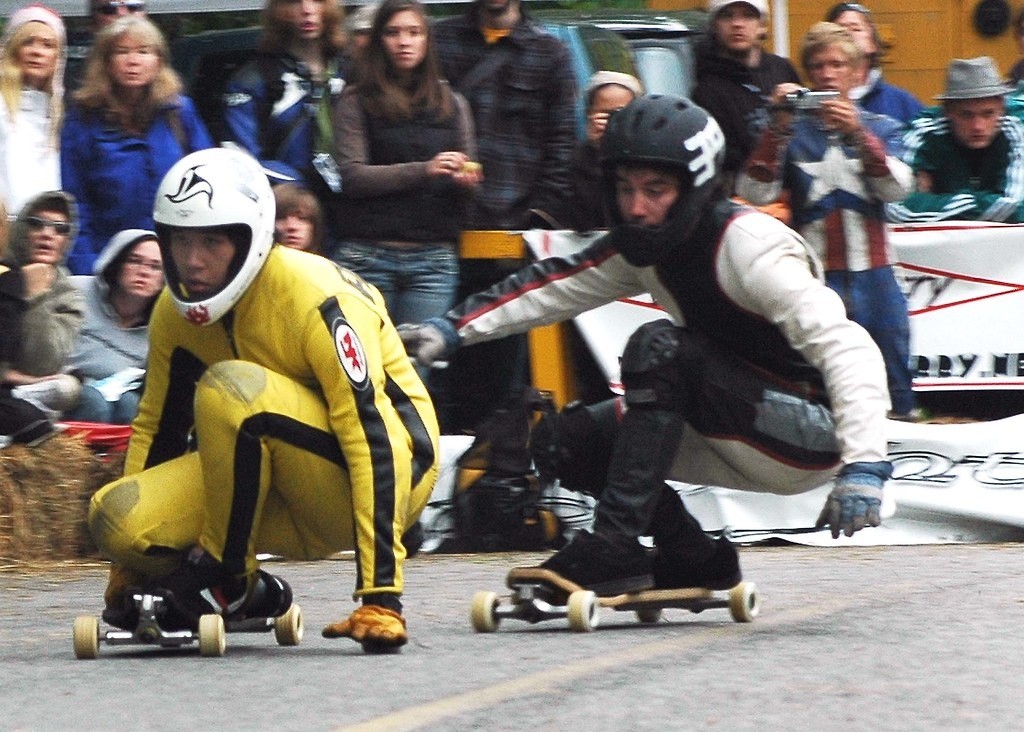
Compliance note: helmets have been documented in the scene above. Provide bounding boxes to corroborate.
[596,90,727,268]
[148,140,277,328]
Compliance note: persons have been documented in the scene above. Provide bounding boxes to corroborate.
[67,229,167,424]
[396,91,892,591]
[0,3,64,222]
[270,180,323,255]
[823,4,934,129]
[217,0,361,194]
[0,190,82,418]
[86,146,441,646]
[326,0,484,357]
[580,70,650,217]
[428,0,579,431]
[736,20,926,420]
[687,0,804,198]
[68,0,200,104]
[883,56,1024,223]
[60,19,216,274]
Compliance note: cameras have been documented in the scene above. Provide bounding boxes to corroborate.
[784,89,840,109]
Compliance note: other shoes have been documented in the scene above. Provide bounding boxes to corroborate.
[98,553,292,627]
[635,527,743,591]
[528,528,656,596]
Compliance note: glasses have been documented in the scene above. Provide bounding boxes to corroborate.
[121,256,163,275]
[24,217,74,238]
[836,2,870,13]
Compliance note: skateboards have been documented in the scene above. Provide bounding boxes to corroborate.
[469,566,763,633]
[70,591,306,657]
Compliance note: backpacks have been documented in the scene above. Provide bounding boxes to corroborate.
[452,388,561,552]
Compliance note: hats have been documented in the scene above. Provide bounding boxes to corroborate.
[702,0,771,25]
[1,3,68,97]
[929,55,1017,100]
[583,69,643,102]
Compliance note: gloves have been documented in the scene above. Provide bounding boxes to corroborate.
[392,318,461,367]
[813,457,896,540]
[321,601,410,648]
[100,561,136,609]
